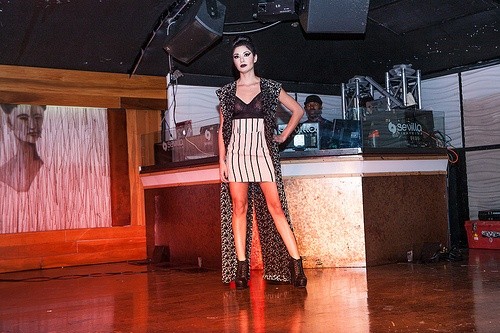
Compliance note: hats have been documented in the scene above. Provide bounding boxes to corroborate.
[304,95,322,106]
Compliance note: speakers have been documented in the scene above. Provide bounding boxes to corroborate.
[162,0,226,64]
[296,0,370,33]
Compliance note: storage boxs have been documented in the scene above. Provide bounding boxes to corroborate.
[464,220,500,249]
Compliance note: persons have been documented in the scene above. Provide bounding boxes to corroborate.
[303,95,333,124]
[0,101,46,193]
[215,37,308,289]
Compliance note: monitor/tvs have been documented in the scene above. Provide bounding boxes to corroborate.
[277,122,321,151]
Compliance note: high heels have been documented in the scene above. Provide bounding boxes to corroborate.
[288,256,307,288]
[235,257,249,287]
